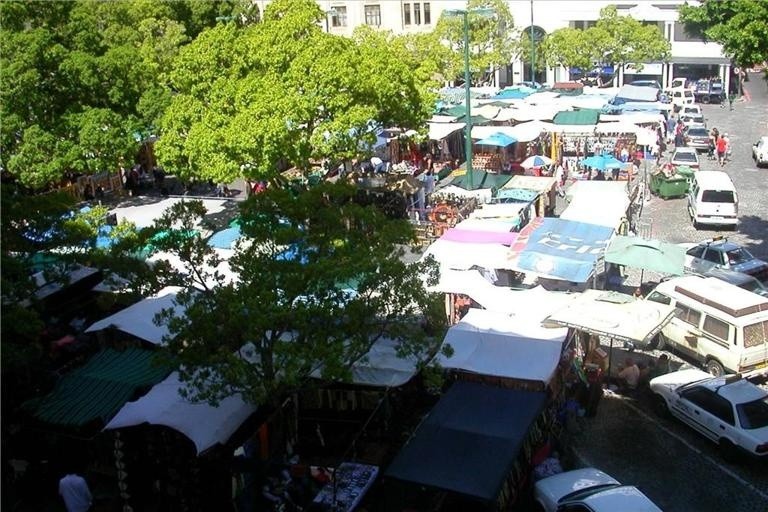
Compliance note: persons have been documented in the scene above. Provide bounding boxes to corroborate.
[633,287,643,300]
[655,104,730,167]
[82,167,139,206]
[370,156,386,173]
[216,179,267,200]
[425,153,434,176]
[154,165,168,196]
[574,142,643,181]
[555,159,565,197]
[528,451,567,489]
[614,353,673,393]
[424,191,484,244]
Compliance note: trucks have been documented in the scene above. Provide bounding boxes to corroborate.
[666,77,724,104]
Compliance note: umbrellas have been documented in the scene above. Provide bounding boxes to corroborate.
[519,154,552,168]
[474,132,518,163]
[605,234,689,288]
[540,289,683,389]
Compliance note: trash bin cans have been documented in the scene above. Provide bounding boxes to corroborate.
[647,166,694,201]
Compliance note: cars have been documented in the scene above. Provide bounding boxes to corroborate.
[533,467,663,512]
[669,147,700,172]
[752,136,768,167]
[678,104,714,152]
[675,235,768,296]
[649,368,768,464]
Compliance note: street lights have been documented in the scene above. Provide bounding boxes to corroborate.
[443,6,498,191]
[325,11,336,33]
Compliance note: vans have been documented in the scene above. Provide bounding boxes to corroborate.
[630,80,662,91]
[687,171,738,231]
[660,88,696,111]
[643,275,768,380]
